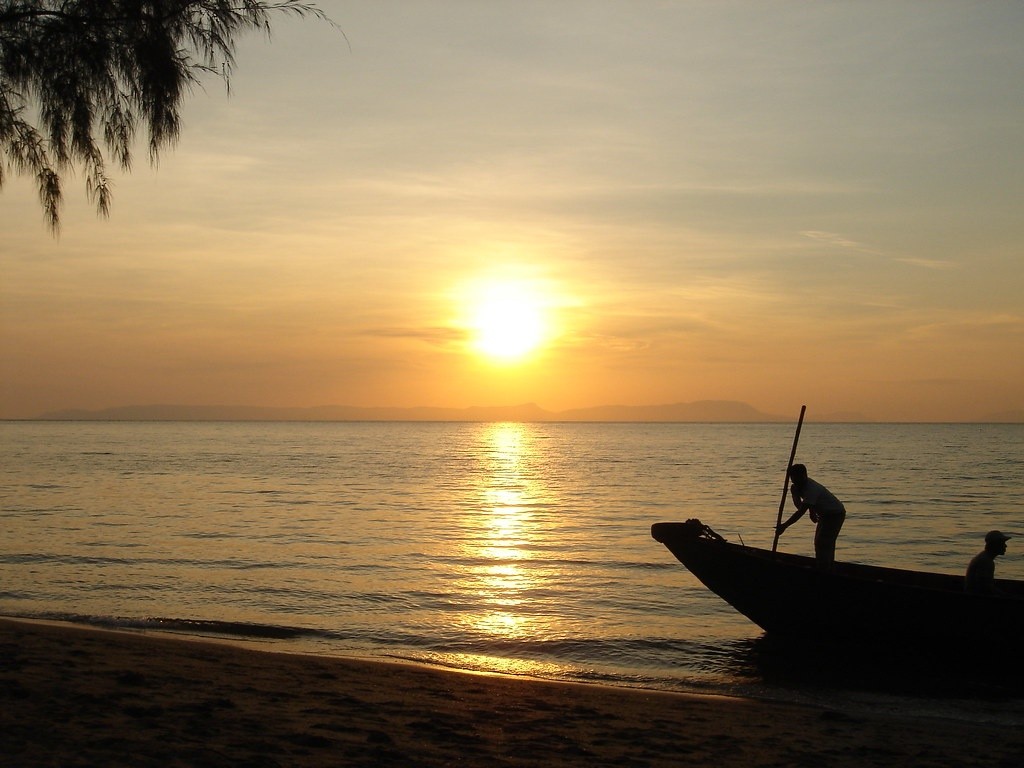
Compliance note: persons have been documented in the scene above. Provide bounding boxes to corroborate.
[966,530,1012,579]
[775,464,846,562]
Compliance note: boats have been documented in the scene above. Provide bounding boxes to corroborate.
[650,518,1024,687]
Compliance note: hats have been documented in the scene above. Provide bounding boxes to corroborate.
[985,530,1012,546]
[791,464,806,474]
